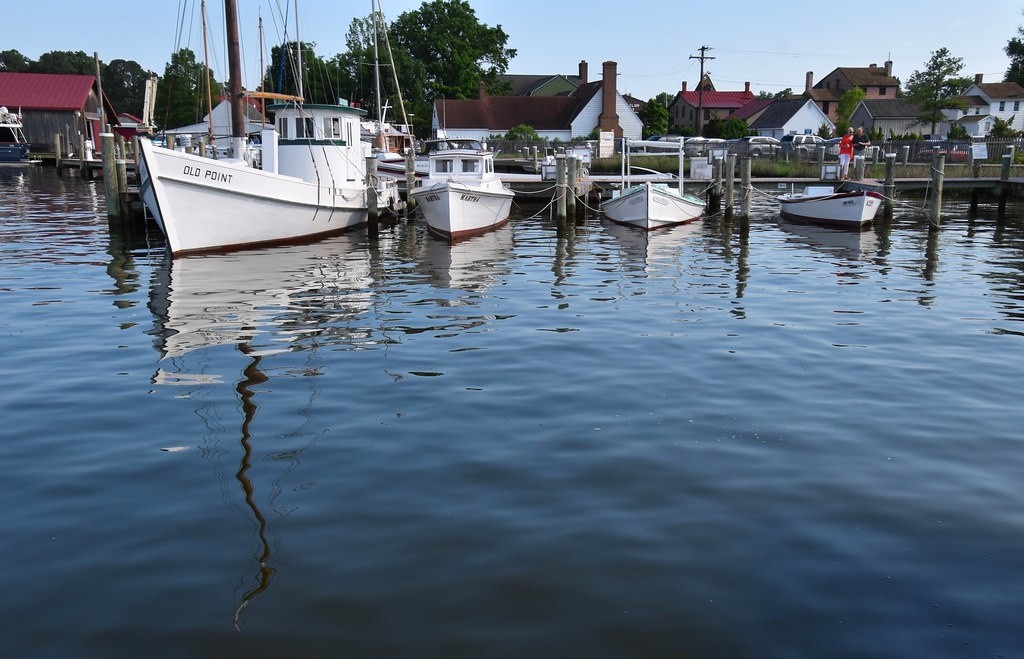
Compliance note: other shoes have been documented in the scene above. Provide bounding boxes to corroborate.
[844,176,848,179]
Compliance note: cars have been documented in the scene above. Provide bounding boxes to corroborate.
[682,136,726,160]
[917,142,970,164]
[715,139,743,163]
[734,136,781,158]
[644,136,692,153]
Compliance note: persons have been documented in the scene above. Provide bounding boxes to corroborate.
[1014,133,1024,151]
[838,127,854,180]
[253,136,260,144]
[851,128,871,180]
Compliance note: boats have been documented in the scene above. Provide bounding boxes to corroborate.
[409,137,515,244]
[601,211,705,283]
[778,214,877,261]
[149,215,397,367]
[366,132,685,200]
[777,185,886,229]
[0,140,44,169]
[137,1,407,259]
[597,139,707,230]
[413,219,516,310]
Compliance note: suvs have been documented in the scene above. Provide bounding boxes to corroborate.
[779,133,827,161]
[826,136,881,161]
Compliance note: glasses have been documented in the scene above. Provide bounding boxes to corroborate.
[850,132,854,134]
